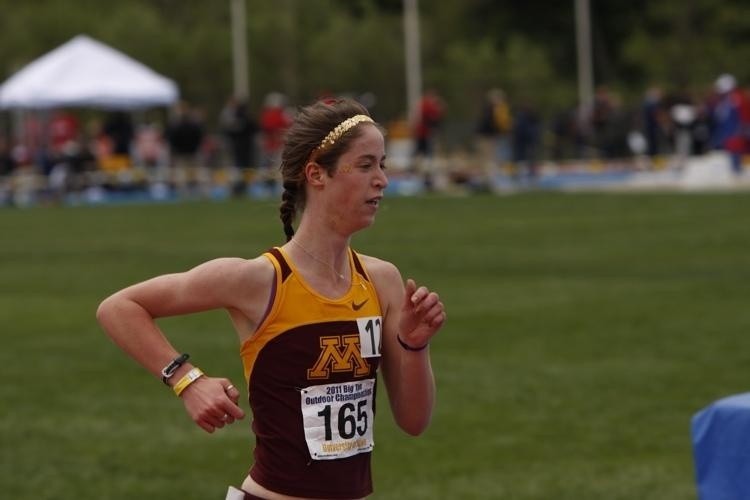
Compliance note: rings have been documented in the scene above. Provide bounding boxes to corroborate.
[218,413,231,423]
[224,382,236,391]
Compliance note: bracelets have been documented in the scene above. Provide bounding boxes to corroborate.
[155,350,196,383]
[394,334,430,352]
[169,369,202,397]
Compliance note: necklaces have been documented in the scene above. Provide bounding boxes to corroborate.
[294,234,353,281]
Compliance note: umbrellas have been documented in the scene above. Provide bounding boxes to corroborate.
[2,33,178,113]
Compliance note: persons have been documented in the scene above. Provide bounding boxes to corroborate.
[90,92,449,499]
[414,72,748,192]
[1,91,296,208]
